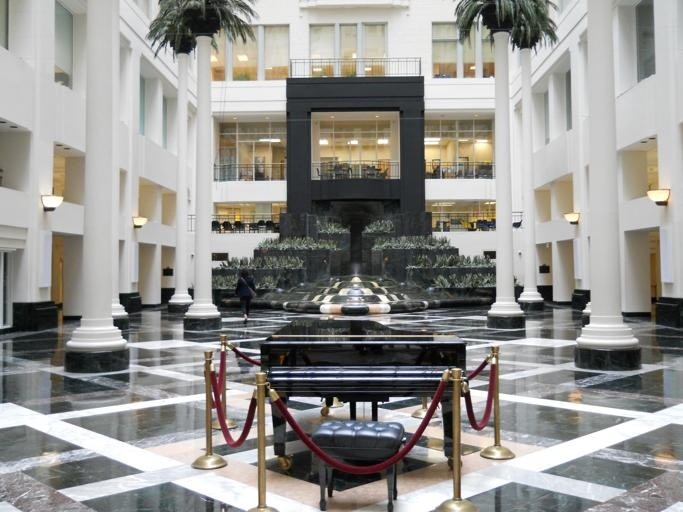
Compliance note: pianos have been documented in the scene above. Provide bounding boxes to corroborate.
[260,318,466,471]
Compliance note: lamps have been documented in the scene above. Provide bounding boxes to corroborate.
[41,195,65,212]
[564,212,579,226]
[134,217,147,227]
[646,188,670,208]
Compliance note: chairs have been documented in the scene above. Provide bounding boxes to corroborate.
[314,156,385,180]
[210,217,279,235]
[476,216,496,232]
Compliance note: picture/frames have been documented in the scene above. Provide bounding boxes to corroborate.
[432,159,440,169]
[459,157,469,172]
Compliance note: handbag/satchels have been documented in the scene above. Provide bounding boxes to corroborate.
[247,286,257,297]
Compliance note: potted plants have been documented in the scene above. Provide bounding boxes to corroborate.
[508,16,558,49]
[148,4,198,58]
[183,0,258,35]
[454,1,554,33]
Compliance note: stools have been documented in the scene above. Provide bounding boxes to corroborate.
[309,419,406,512]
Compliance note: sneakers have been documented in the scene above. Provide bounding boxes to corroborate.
[244,317,248,324]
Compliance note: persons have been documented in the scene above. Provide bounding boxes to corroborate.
[235,269,256,324]
[232,336,259,375]
